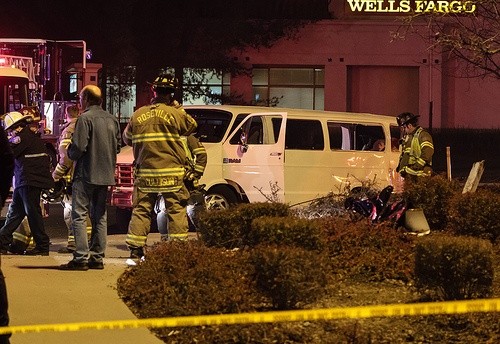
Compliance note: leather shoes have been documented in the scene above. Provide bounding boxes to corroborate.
[0,239,13,252]
[26,248,49,256]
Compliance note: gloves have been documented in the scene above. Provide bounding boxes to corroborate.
[184,169,203,181]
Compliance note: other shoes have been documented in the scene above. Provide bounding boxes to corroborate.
[58,247,74,254]
[131,248,144,257]
[8,242,25,254]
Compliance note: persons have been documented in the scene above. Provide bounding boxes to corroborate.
[0,75,208,271]
[396,112,435,184]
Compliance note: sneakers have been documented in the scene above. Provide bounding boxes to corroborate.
[87,259,104,268]
[61,259,89,269]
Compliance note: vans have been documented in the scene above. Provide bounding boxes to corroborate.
[107,104,405,231]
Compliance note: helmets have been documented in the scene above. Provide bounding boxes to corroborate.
[4,111,32,130]
[152,75,178,88]
[396,112,421,128]
[41,177,66,202]
